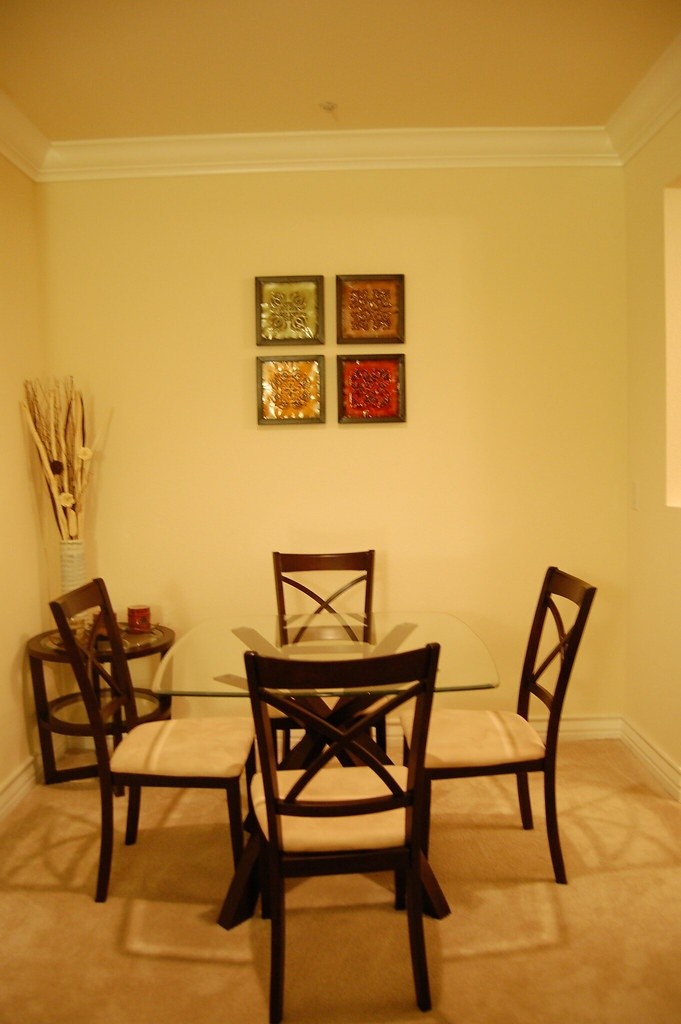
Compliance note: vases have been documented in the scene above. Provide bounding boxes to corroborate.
[61,539,87,634]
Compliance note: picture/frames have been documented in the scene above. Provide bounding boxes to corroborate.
[255,275,325,346]
[256,355,325,425]
[336,274,405,344]
[337,354,406,423]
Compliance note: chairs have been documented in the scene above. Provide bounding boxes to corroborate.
[50,578,257,902]
[245,642,442,1024]
[400,566,598,885]
[267,550,386,764]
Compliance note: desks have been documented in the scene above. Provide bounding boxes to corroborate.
[151,611,499,930]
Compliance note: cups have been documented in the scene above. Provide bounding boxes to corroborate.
[127,605,151,632]
[69,618,86,640]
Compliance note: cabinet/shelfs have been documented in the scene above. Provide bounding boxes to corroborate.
[28,622,175,785]
